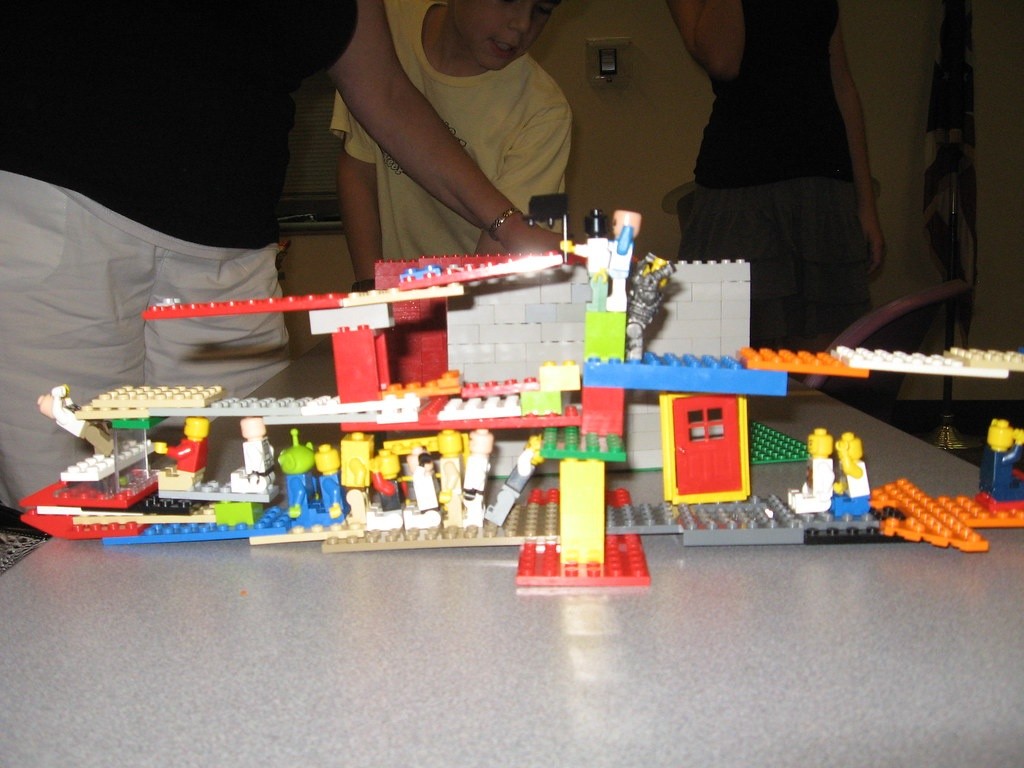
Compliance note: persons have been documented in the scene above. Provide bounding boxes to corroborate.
[489,208,523,241]
[664,0,887,352]
[0,0,564,516]
[330,1,573,291]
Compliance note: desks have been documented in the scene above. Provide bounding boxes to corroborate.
[0,333,1024,768]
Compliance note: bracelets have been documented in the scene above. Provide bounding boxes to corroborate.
[350,279,376,292]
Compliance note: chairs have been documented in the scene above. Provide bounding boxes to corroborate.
[802,277,972,422]
